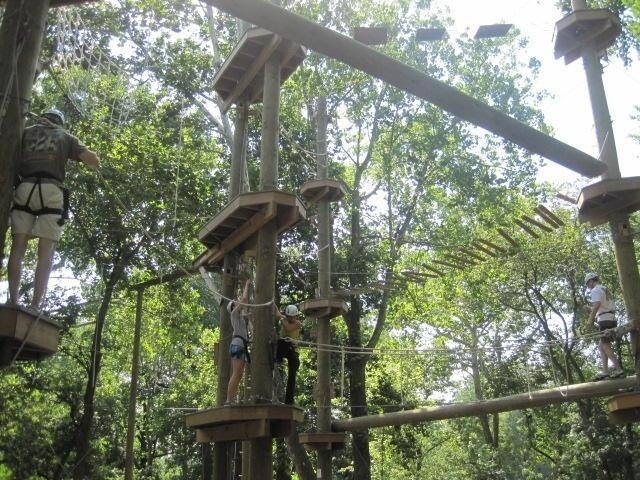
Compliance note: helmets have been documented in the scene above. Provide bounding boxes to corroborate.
[285,305,299,316]
[584,272,598,284]
[226,300,233,313]
[40,108,64,125]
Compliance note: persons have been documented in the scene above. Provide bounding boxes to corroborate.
[6,108,100,310]
[274,302,303,405]
[225,279,254,406]
[583,270,624,378]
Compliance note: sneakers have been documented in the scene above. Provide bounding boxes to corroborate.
[610,370,625,379]
[591,373,609,381]
[223,400,238,407]
[28,306,41,314]
